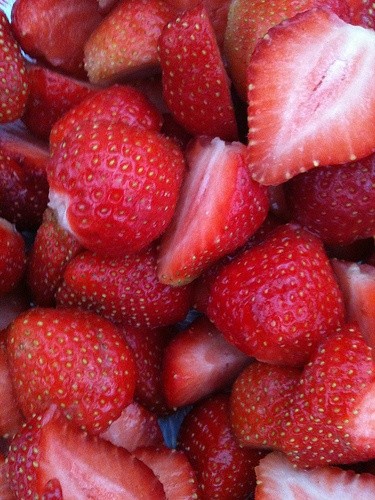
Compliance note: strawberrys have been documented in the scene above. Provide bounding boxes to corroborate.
[0,0,375,500]
